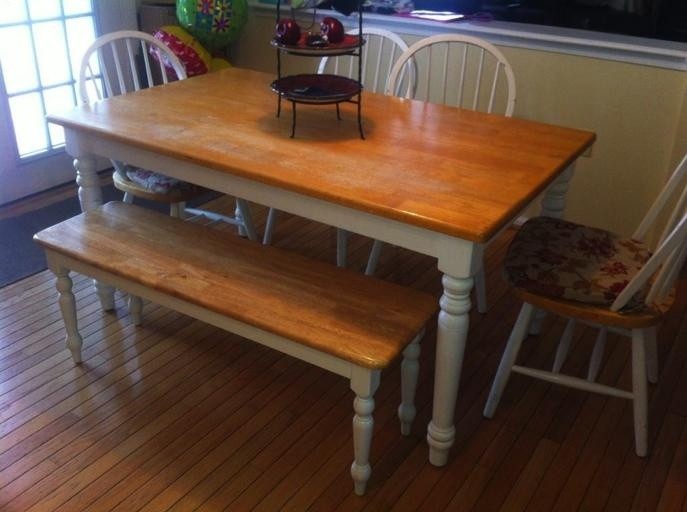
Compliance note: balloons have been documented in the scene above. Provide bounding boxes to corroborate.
[149,24,212,80]
[175,0,247,45]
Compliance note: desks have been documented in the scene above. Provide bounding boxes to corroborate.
[46,66,597,468]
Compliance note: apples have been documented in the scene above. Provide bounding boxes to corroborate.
[318,16,345,42]
[274,19,300,43]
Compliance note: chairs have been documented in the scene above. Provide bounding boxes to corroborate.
[482,153,687,459]
[365,33,518,315]
[263,27,419,267]
[79,30,257,240]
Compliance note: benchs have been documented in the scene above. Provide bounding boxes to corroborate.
[31,200,440,497]
